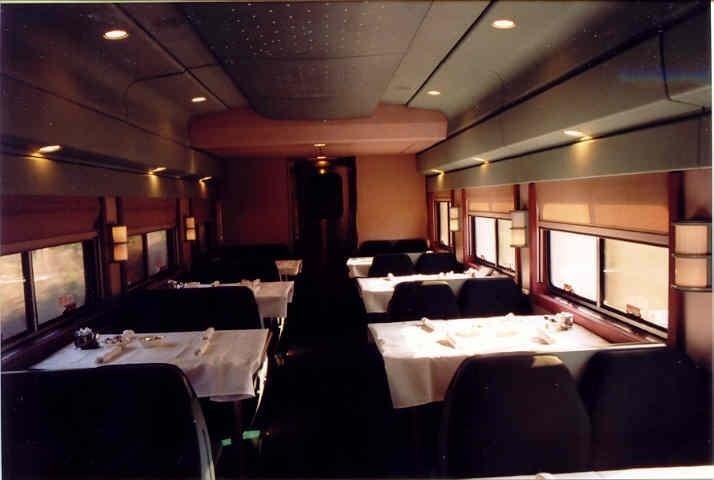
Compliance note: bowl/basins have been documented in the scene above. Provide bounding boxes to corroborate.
[139,336,162,348]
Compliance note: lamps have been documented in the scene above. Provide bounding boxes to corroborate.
[449,207,461,232]
[671,220,713,292]
[509,209,528,247]
[185,217,196,240]
[112,226,129,262]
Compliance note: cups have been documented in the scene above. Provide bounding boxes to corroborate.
[74,328,100,350]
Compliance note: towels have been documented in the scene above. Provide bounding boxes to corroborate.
[533,328,552,344]
[202,327,214,340]
[95,343,126,363]
[121,330,135,343]
[437,332,456,347]
[192,340,209,357]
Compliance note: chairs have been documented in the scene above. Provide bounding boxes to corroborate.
[79,364,216,480]
[2,369,79,480]
[576,346,713,471]
[428,357,593,480]
